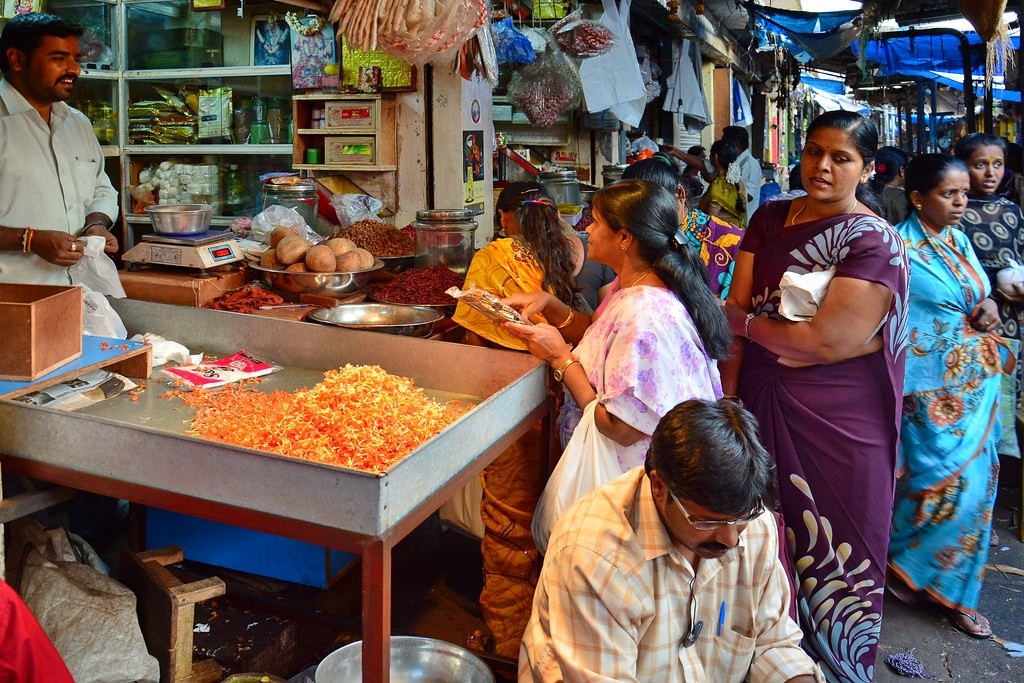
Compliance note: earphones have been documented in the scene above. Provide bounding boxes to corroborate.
[683,621,701,648]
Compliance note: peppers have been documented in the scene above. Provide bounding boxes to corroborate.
[551,17,615,59]
[365,263,467,305]
[513,81,570,126]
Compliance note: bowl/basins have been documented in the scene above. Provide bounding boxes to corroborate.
[368,294,457,318]
[248,261,385,298]
[144,204,213,234]
[315,635,496,683]
[306,303,444,338]
[578,190,595,202]
[377,255,415,273]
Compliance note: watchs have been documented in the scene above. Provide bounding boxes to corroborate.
[553,357,580,382]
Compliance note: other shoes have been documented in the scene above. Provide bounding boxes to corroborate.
[989,528,1000,545]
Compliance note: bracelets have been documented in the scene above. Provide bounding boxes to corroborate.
[19,227,34,253]
[743,312,757,344]
[79,221,108,237]
[555,304,574,329]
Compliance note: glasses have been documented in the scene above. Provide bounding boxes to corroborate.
[666,488,765,531]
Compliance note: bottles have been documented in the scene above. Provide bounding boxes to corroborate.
[311,109,325,129]
[261,183,319,232]
[411,208,478,277]
[469,134,480,164]
[466,166,474,201]
[602,163,631,186]
[226,164,244,215]
[538,169,580,207]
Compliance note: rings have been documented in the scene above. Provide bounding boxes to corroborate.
[975,304,981,308]
[71,242,77,252]
[985,320,990,326]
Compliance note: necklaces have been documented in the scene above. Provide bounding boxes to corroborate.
[614,268,653,295]
[791,197,858,227]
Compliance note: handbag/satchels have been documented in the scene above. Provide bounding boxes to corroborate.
[997,258,1024,295]
[250,204,307,245]
[995,337,1020,458]
[778,268,889,367]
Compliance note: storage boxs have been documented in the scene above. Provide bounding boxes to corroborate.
[1,282,83,382]
[144,505,365,590]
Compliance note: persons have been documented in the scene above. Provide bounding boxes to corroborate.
[448,110,1024,683]
[0,11,118,295]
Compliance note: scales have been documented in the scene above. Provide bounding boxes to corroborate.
[120,231,246,279]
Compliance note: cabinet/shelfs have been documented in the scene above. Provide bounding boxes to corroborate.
[41,0,305,269]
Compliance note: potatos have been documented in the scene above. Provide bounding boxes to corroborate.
[261,226,375,273]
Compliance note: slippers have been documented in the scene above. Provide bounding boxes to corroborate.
[464,630,517,683]
[949,608,992,637]
[885,564,910,600]
